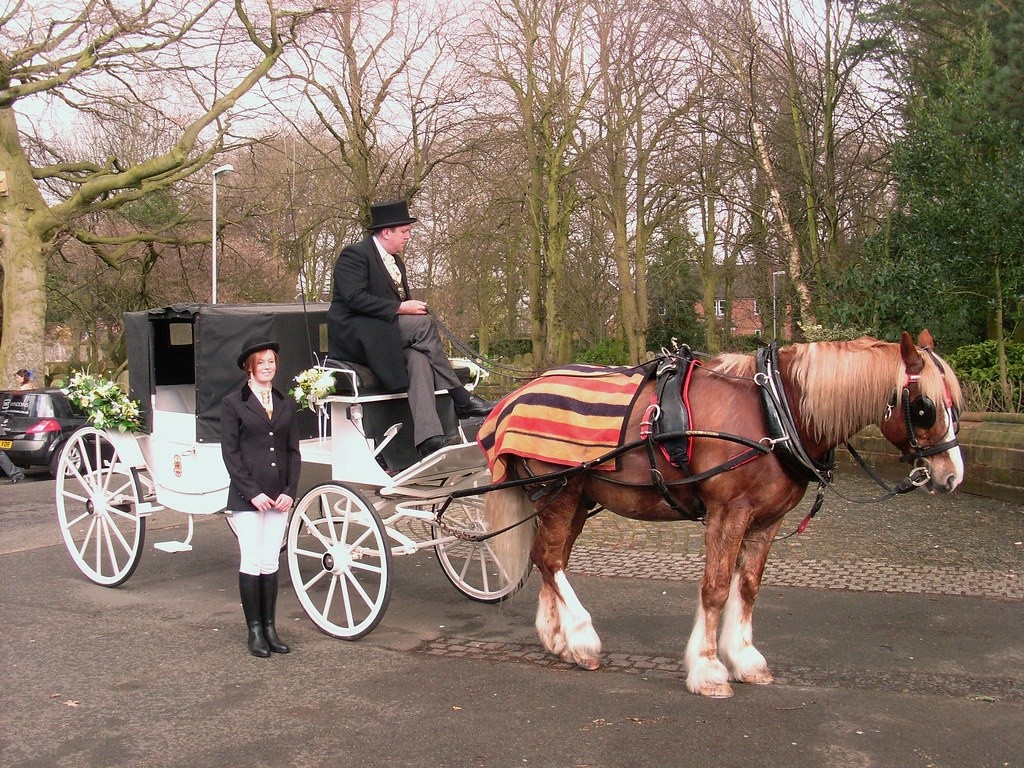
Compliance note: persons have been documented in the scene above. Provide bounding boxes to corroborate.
[327,200,492,458]
[0,447,25,485]
[16,369,39,391]
[220,336,301,658]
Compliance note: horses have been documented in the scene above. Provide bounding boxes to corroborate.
[480,327,966,700]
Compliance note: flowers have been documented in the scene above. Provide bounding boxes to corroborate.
[59,363,146,434]
[287,351,337,414]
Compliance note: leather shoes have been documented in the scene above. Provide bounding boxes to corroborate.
[454,393,498,420]
[418,435,461,460]
[8,473,25,484]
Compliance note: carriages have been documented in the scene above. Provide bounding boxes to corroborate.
[55,306,965,697]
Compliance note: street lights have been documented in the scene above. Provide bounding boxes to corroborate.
[773,271,786,340]
[212,164,235,304]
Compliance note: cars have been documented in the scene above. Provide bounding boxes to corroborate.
[0,387,102,479]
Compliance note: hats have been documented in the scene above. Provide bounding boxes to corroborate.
[365,199,418,230]
[236,336,280,370]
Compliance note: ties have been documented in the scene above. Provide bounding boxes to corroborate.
[262,389,271,419]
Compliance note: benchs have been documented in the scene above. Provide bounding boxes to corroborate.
[155,384,196,415]
[313,358,480,403]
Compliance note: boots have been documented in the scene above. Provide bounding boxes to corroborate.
[239,569,291,657]
